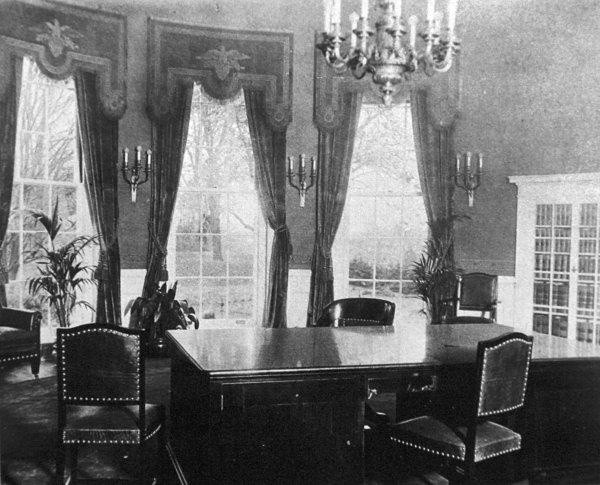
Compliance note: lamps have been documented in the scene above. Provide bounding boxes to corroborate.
[316,0,466,108]
[451,146,488,210]
[285,148,323,208]
[121,138,153,203]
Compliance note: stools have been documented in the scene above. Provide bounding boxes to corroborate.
[1,305,46,382]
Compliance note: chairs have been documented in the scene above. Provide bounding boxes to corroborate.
[382,331,534,483]
[310,297,396,328]
[440,273,497,323]
[52,323,171,485]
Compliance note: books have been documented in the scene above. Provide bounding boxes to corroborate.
[531,204,599,345]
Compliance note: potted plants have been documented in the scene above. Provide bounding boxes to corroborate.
[120,280,204,360]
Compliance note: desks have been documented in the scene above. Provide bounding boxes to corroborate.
[156,320,598,483]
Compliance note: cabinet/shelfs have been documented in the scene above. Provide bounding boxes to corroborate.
[505,168,598,347]
